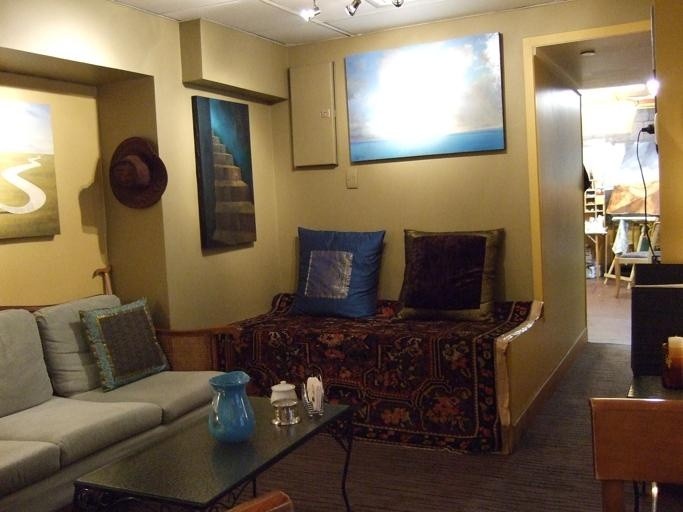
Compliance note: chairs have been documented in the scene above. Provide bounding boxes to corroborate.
[615,222,662,298]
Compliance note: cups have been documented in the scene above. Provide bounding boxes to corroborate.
[271,380,298,404]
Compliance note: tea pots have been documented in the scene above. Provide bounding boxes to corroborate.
[205,371,259,447]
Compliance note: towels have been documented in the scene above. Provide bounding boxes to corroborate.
[612,220,629,258]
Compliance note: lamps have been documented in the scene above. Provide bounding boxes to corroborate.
[301,7,319,21]
[392,0,403,7]
[345,0,361,15]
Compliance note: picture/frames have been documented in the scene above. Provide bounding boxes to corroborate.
[345,33,506,164]
[192,96,257,249]
[1,99,60,239]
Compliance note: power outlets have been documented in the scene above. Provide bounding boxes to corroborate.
[652,108,660,151]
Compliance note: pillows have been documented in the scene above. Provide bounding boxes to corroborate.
[292,227,387,324]
[392,229,501,322]
[78,297,168,391]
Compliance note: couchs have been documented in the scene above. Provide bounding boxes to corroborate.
[217,293,545,456]
[1,293,226,512]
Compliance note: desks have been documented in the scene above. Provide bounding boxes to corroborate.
[603,217,658,288]
[590,373,683,512]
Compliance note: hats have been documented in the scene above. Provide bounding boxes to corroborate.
[110,137,168,210]
